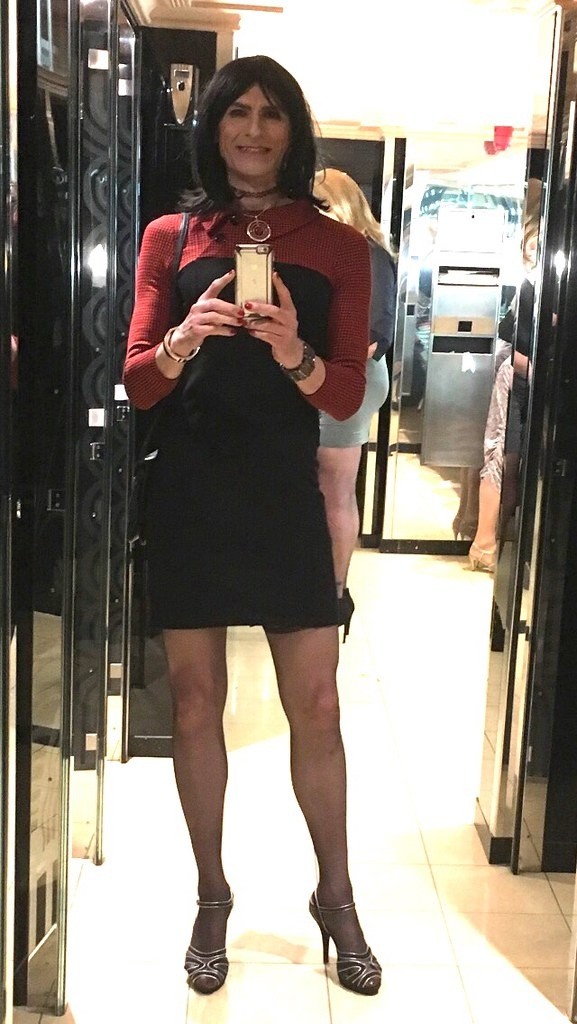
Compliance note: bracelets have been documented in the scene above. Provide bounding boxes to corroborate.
[280,341,316,383]
[164,327,201,363]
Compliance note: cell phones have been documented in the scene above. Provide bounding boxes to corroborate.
[234,244,273,322]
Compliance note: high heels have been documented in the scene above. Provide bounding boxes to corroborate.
[186,887,235,994]
[339,587,355,643]
[468,541,498,573]
[452,516,478,541]
[309,890,382,994]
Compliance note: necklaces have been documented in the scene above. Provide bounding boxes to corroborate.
[240,203,270,242]
[231,186,279,199]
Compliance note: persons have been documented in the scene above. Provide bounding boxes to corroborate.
[467,176,545,575]
[123,57,382,996]
[310,168,396,636]
